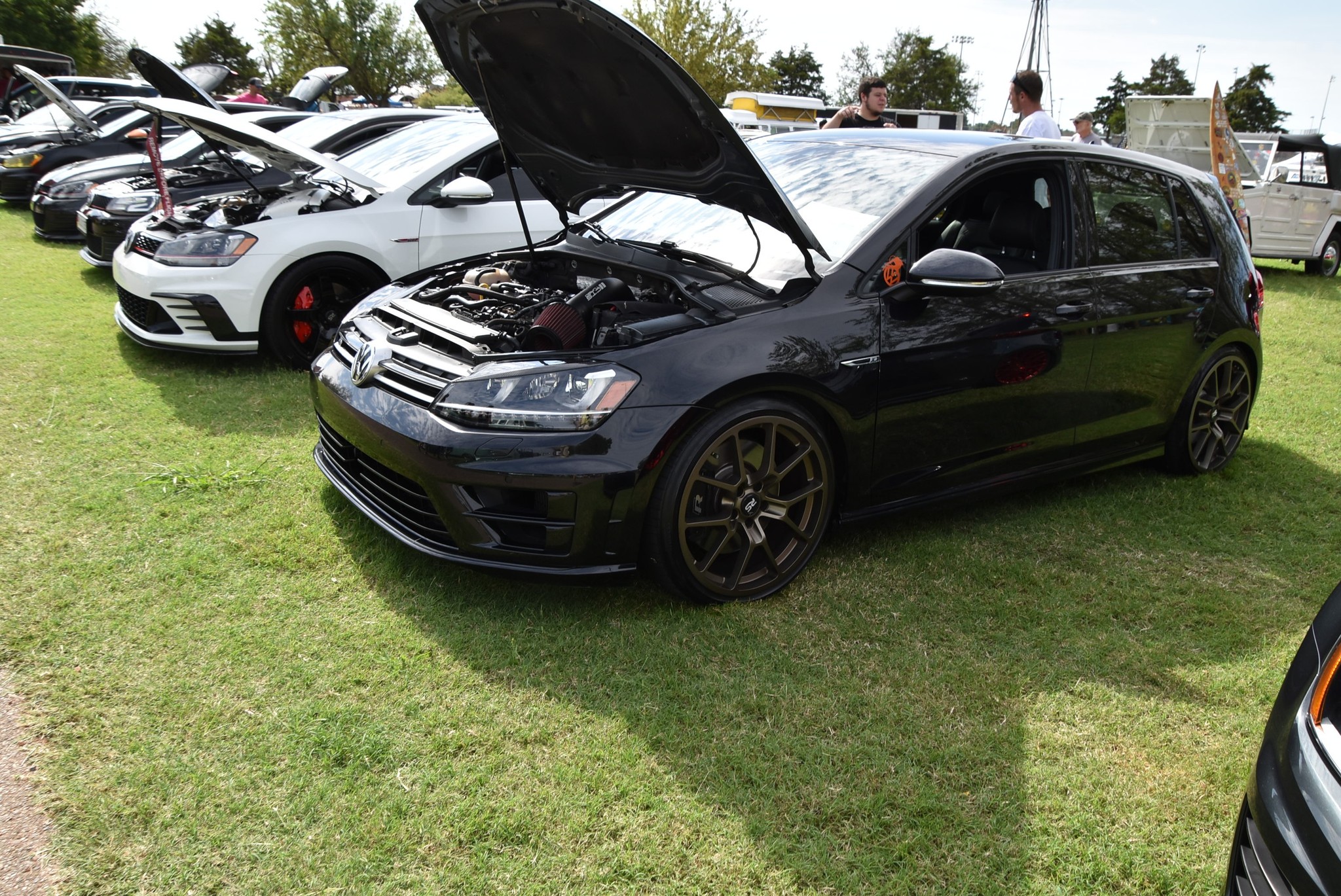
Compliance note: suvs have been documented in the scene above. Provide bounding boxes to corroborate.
[1126,95,1341,278]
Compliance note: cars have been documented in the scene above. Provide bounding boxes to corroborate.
[310,0,1266,609]
[0,48,632,373]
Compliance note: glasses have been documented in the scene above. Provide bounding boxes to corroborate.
[1010,73,1029,95]
[256,86,263,88]
[1072,121,1082,124]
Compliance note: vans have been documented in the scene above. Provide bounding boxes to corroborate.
[736,120,817,136]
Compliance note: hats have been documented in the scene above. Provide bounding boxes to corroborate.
[249,78,266,87]
[1069,112,1093,123]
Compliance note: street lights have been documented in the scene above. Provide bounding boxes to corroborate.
[1192,43,1206,97]
[951,34,975,112]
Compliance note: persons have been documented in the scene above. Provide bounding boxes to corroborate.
[1070,111,1102,145]
[225,77,268,105]
[821,75,901,128]
[1008,69,1061,139]
[819,120,827,129]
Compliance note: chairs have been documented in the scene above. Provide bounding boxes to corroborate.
[1099,202,1171,266]
[936,181,1007,255]
[984,199,1044,275]
